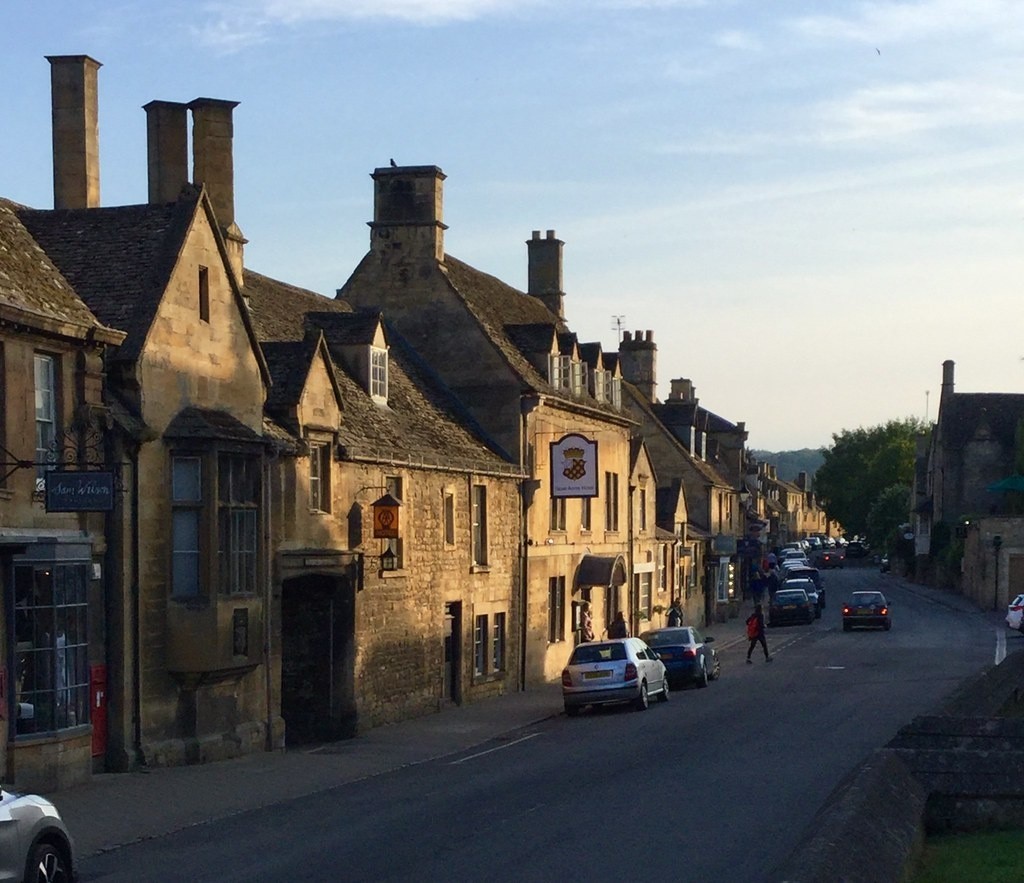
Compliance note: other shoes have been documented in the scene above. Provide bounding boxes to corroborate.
[745,659,752,664]
[765,657,774,662]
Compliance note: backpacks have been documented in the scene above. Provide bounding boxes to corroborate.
[747,615,759,639]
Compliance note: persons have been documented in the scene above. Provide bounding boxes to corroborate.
[610,611,625,638]
[745,604,774,663]
[750,550,782,610]
[580,601,594,642]
[665,597,683,628]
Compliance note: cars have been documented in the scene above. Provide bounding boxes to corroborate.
[561,637,671,716]
[634,625,720,688]
[815,549,845,570]
[770,536,872,608]
[842,590,892,631]
[767,588,815,627]
[0,784,78,883]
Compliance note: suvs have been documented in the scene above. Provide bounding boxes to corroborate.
[777,576,822,618]
[1006,594,1024,634]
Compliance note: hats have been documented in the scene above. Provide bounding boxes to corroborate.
[753,604,763,609]
